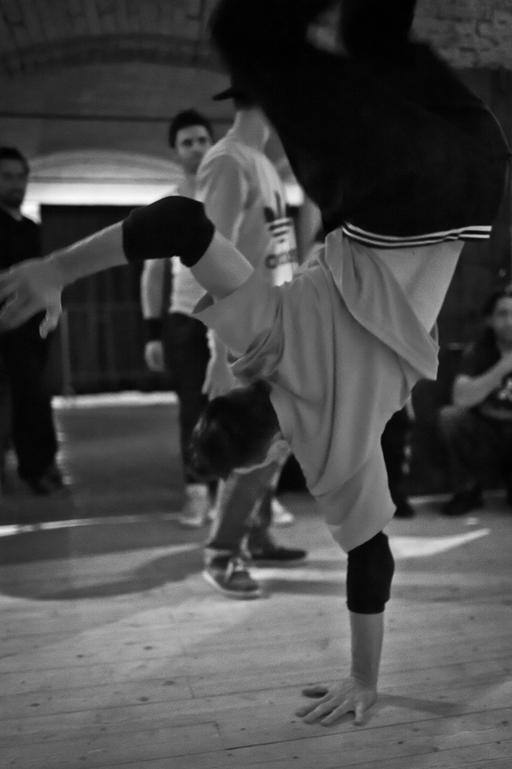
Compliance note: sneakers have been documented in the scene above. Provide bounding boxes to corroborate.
[178,484,213,528]
[269,496,293,527]
[201,556,260,599]
[439,495,483,515]
[22,461,63,494]
[242,545,308,566]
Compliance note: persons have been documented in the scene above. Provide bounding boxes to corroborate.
[197,67,314,600]
[2,102,512,533]
[2,0,511,728]
